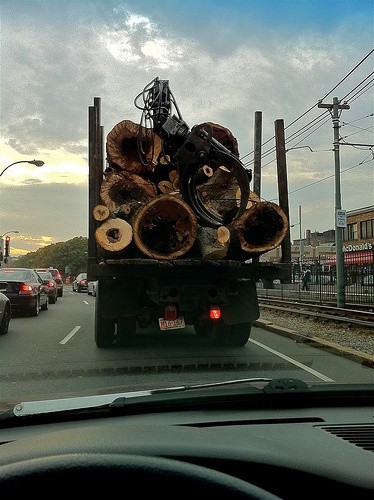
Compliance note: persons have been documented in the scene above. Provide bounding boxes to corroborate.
[301,266,311,291]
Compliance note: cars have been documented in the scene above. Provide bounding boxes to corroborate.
[86,280,98,297]
[0,282,11,335]
[72,273,87,292]
[48,268,65,297]
[0,268,49,317]
[18,269,61,304]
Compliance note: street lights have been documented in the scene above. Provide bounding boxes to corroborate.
[290,204,303,278]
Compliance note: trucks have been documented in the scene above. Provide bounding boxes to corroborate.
[85,96,292,349]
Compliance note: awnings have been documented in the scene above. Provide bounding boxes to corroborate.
[322,250,374,265]
